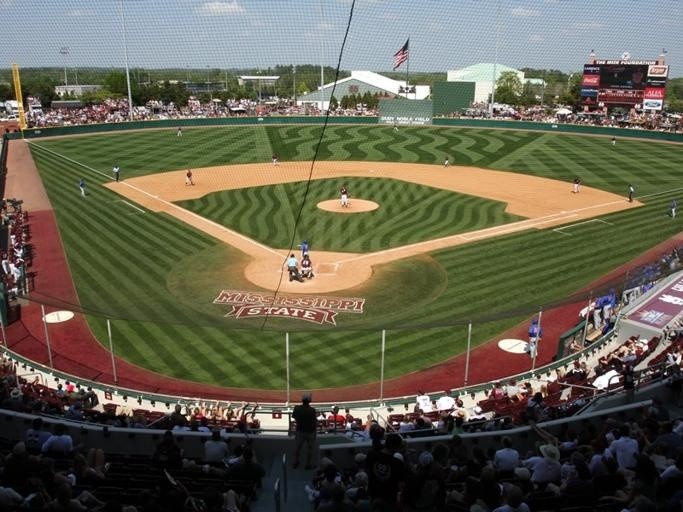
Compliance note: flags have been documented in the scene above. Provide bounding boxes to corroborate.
[392,39,409,71]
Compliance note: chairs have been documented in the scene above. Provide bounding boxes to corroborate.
[1,352,262,512]
[478,335,683,408]
[318,409,682,511]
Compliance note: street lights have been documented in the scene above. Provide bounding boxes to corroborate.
[566,73,573,92]
[313,23,330,116]
[206,65,209,84]
[185,65,191,81]
[72,68,78,85]
[540,68,551,107]
[59,47,68,87]
[291,64,297,106]
[256,70,263,118]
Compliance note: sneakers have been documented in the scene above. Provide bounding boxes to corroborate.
[304,485,316,503]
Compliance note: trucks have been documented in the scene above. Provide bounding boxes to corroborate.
[0,98,45,124]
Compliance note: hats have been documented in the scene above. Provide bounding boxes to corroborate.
[302,391,312,403]
[539,444,561,464]
[514,467,532,480]
